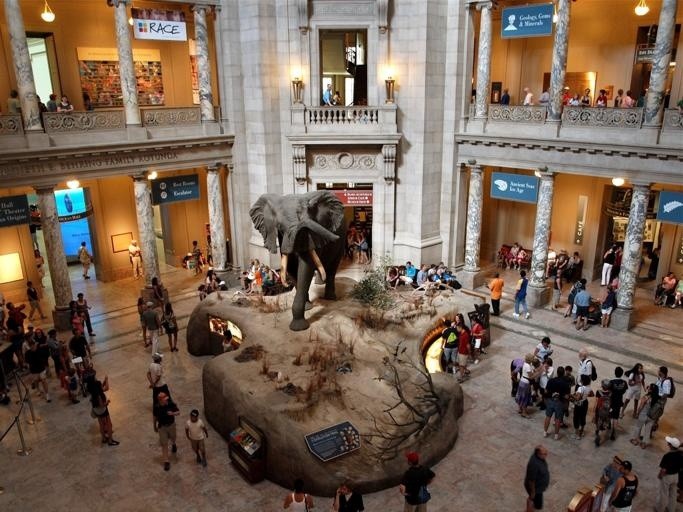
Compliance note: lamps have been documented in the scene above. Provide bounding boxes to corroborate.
[37,0,58,25]
[384,75,395,106]
[289,76,303,106]
[610,177,626,187]
[634,0,649,18]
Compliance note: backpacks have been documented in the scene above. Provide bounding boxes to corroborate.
[646,394,664,420]
[597,390,611,416]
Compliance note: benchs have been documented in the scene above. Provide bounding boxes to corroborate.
[380,264,415,286]
[496,242,531,272]
[243,264,273,292]
[652,275,682,306]
[546,253,584,283]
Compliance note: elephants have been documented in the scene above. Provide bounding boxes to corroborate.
[250,189,349,332]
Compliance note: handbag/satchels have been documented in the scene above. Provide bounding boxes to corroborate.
[568,396,582,407]
[469,336,475,346]
[660,377,675,398]
[528,377,535,385]
[92,407,106,415]
[585,360,597,381]
[168,320,174,330]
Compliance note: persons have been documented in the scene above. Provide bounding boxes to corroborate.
[1,204,120,446]
[182,223,228,301]
[129,240,144,281]
[147,352,208,471]
[441,313,487,384]
[341,226,372,265]
[5,87,93,136]
[283,478,314,511]
[511,336,672,449]
[137,277,179,362]
[385,261,462,290]
[470,82,682,121]
[333,478,365,511]
[524,444,550,510]
[222,330,240,353]
[330,91,342,106]
[235,258,293,296]
[322,84,333,107]
[636,244,682,309]
[485,242,622,331]
[654,436,682,511]
[600,454,640,511]
[399,451,435,511]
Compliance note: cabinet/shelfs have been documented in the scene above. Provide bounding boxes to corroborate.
[224,414,266,484]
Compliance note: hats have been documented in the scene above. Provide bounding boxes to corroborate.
[151,353,163,360]
[664,436,679,448]
[190,410,198,417]
[146,302,153,306]
[613,455,631,469]
[405,453,418,462]
[157,393,168,401]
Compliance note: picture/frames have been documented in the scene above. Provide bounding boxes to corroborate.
[0,250,25,285]
[108,231,136,254]
